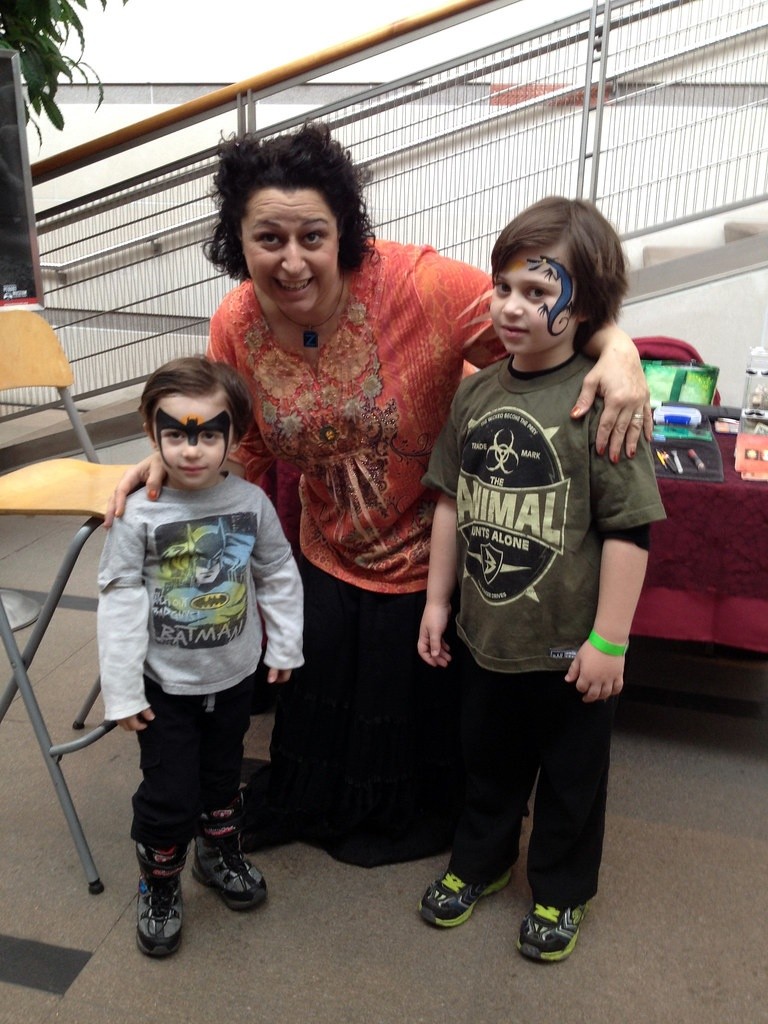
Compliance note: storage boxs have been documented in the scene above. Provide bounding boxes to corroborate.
[654,407,702,430]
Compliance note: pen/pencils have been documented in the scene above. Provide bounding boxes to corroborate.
[656,448,677,473]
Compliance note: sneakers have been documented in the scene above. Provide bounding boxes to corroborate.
[516,900,589,962]
[134,842,189,957]
[418,863,513,928]
[191,791,268,909]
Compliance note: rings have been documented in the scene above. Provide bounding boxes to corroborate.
[634,413,644,421]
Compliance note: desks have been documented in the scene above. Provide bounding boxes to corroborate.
[630,405,768,652]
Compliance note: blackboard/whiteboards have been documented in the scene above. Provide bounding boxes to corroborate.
[0,47,45,312]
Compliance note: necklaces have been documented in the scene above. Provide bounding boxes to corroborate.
[278,273,346,349]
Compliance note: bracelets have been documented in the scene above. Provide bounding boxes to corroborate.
[587,628,629,661]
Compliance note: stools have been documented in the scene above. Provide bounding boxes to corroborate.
[0,309,139,895]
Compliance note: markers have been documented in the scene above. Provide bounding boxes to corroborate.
[688,449,704,468]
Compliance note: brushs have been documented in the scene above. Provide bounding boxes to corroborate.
[670,449,683,474]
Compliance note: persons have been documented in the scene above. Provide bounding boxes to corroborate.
[415,196,666,961]
[97,359,305,958]
[105,121,655,871]
